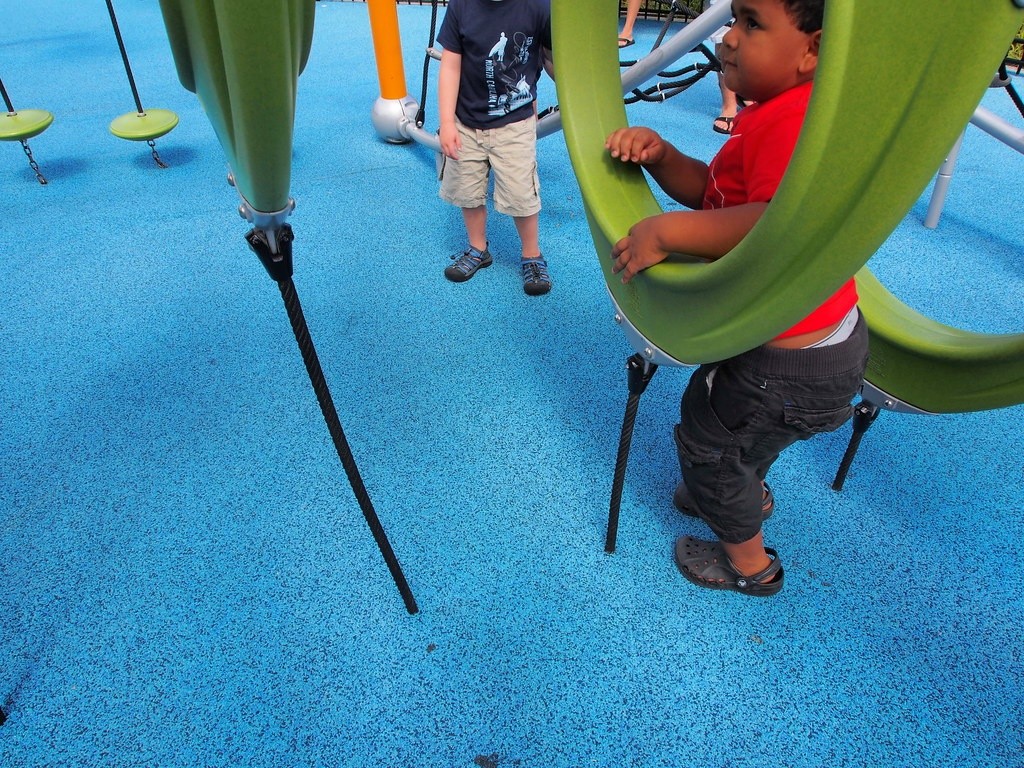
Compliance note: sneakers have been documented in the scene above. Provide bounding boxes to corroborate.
[444,240,493,282]
[521,252,552,295]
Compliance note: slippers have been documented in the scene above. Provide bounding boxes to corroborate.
[618,38,635,48]
[713,117,735,134]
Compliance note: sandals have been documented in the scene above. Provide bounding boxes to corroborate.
[674,536,784,596]
[673,480,775,521]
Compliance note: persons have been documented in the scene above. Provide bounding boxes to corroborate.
[617,0,642,48]
[434,0,556,295]
[603,0,869,597]
[708,18,755,134]
